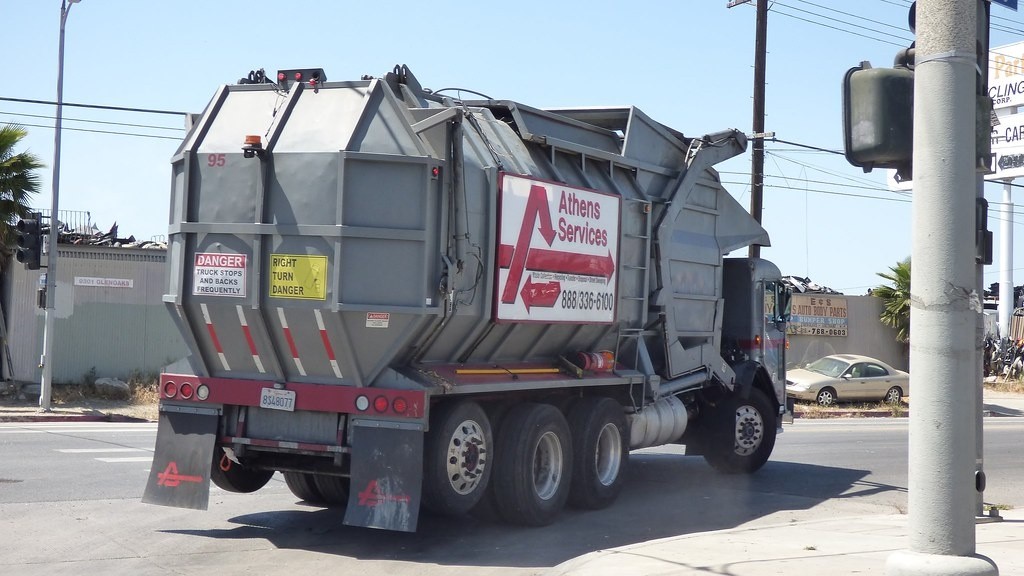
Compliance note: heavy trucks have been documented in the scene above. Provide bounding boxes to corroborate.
[140,63,795,531]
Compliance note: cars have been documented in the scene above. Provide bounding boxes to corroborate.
[784,353,909,406]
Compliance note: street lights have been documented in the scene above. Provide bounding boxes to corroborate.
[39,0,83,411]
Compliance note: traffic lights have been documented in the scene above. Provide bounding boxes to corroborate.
[16,213,42,269]
